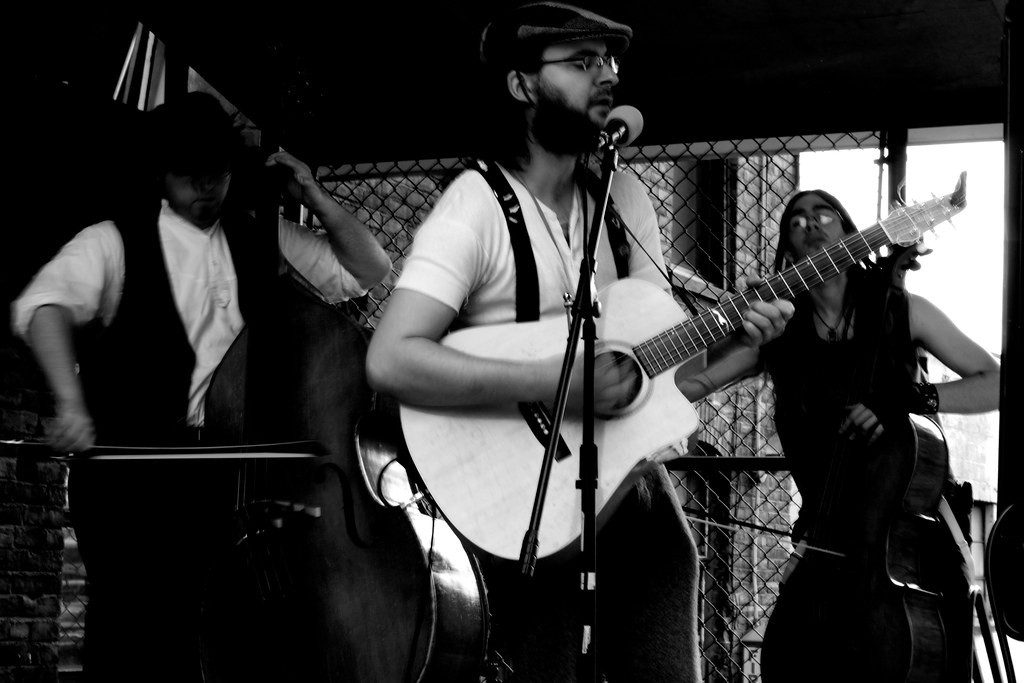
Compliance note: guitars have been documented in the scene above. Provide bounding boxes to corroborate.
[397,170,970,563]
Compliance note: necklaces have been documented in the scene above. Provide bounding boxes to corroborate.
[813,304,845,341]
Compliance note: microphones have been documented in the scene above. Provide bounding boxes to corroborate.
[592,105,643,153]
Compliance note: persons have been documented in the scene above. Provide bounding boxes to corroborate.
[365,0,795,683]
[676,191,999,683]
[12,91,390,683]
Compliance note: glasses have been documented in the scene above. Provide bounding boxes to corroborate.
[789,214,834,231]
[544,55,613,73]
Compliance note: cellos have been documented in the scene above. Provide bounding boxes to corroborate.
[212,85,488,683]
[758,241,944,683]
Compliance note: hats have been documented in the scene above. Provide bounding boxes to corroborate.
[141,90,244,179]
[480,2,632,77]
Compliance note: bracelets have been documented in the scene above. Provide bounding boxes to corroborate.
[914,381,939,415]
